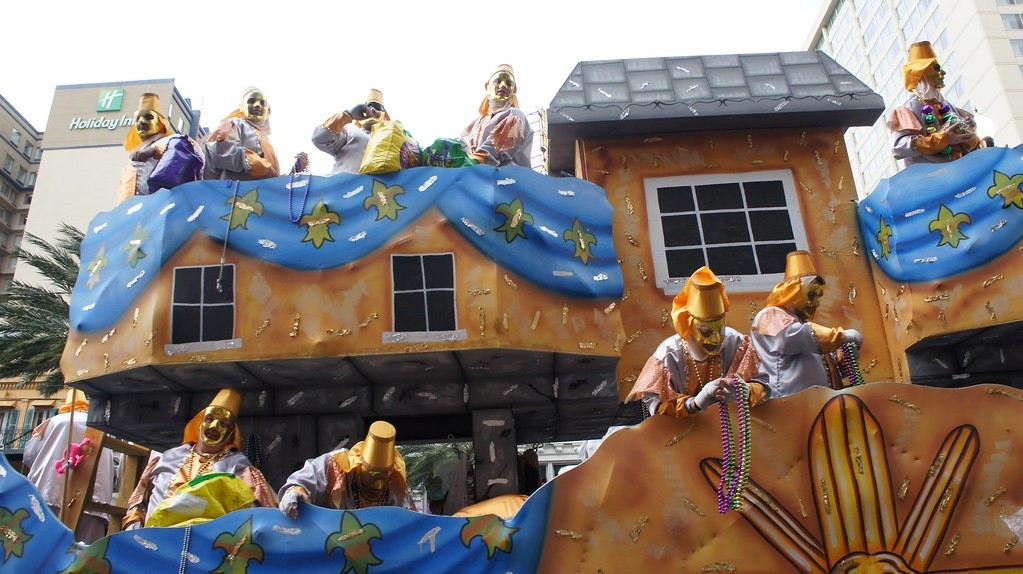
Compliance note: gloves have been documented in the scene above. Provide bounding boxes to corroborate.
[124,521,144,531]
[345,104,369,121]
[844,327,865,350]
[279,484,309,518]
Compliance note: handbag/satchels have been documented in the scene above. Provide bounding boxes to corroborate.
[358,121,423,175]
[421,137,480,168]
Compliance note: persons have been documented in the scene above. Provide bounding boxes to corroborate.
[23,389,114,545]
[121,388,281,532]
[624,266,772,418]
[885,41,979,169]
[123,93,205,195]
[278,421,418,520]
[982,136,994,147]
[201,86,308,180]
[460,64,534,168]
[312,89,391,173]
[750,250,864,398]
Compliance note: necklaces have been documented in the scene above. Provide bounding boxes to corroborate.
[840,344,866,386]
[915,91,954,157]
[351,479,388,509]
[288,155,311,222]
[682,339,714,389]
[718,376,752,514]
[188,444,220,482]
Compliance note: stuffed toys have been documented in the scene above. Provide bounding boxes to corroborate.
[55,438,90,478]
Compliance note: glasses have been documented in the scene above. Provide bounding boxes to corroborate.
[135,114,154,121]
[805,290,824,299]
[367,101,386,112]
[245,98,267,107]
[691,327,724,338]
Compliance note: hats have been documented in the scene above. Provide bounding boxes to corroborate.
[334,421,406,492]
[764,250,826,307]
[355,89,391,127]
[183,385,243,449]
[902,41,938,92]
[57,388,89,414]
[934,64,942,71]
[671,266,730,344]
[220,85,268,131]
[478,64,518,114]
[123,93,176,153]
